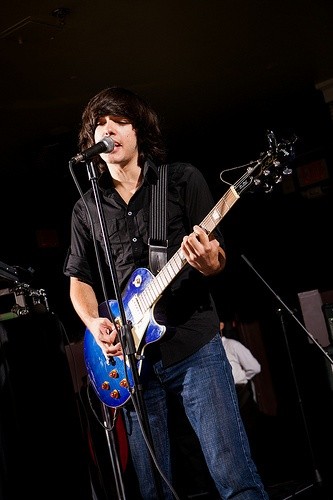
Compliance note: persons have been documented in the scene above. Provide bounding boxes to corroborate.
[218,320,262,409]
[62,85,270,500]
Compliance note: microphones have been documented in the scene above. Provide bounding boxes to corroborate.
[71,137,114,165]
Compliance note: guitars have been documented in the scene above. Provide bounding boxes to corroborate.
[84,131,298,408]
